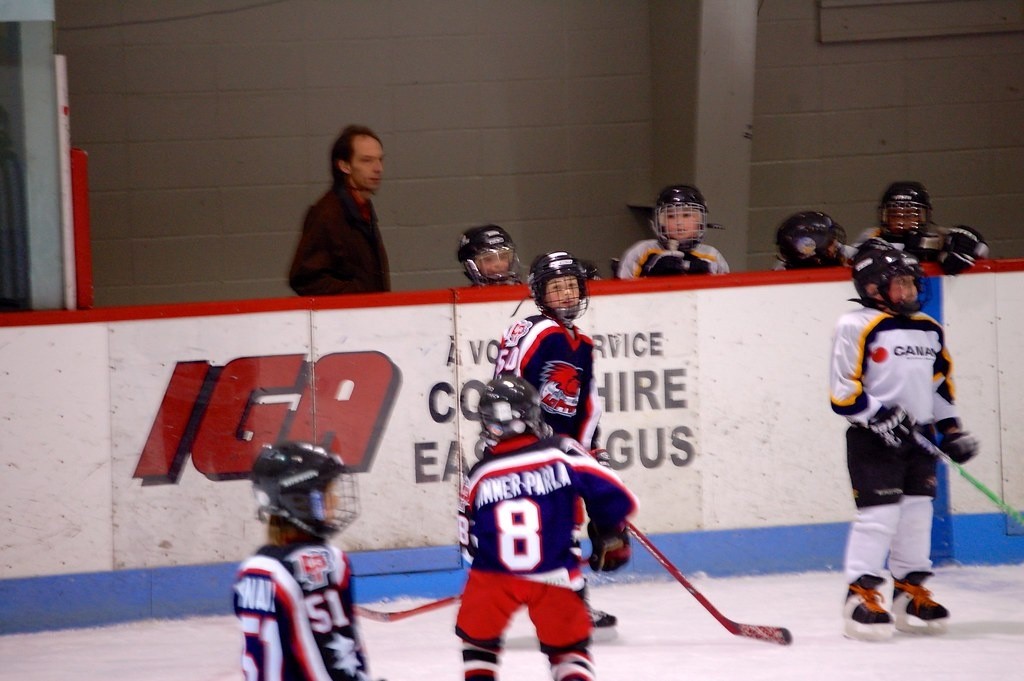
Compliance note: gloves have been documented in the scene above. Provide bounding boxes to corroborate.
[587,520,631,572]
[941,226,981,274]
[940,432,978,464]
[641,251,709,278]
[870,405,913,449]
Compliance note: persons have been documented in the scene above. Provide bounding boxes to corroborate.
[288,125,391,296]
[495,251,619,642]
[232,440,370,680]
[460,224,524,285]
[829,239,978,642]
[775,182,988,275]
[619,184,730,277]
[454,375,638,681]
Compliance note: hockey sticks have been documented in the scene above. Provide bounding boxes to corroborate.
[352,593,463,624]
[623,515,793,646]
[912,427,1024,529]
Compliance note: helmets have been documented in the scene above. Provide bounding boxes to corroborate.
[476,376,542,441]
[854,239,931,315]
[650,185,709,251]
[256,443,358,539]
[458,220,523,287]
[529,249,588,322]
[879,181,933,247]
[775,213,846,265]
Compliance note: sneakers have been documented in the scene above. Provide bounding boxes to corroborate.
[892,571,950,634]
[575,577,617,642]
[841,574,895,642]
